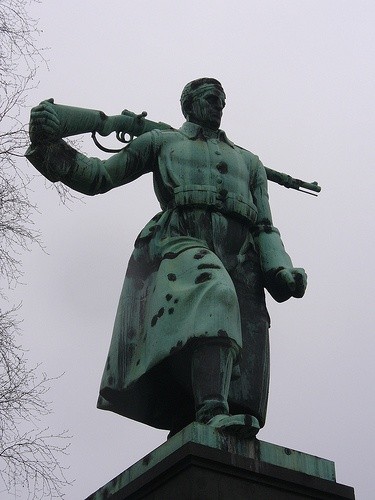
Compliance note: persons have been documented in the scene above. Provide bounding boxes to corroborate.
[24,76,309,437]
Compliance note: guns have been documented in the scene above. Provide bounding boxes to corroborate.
[46,103,321,196]
